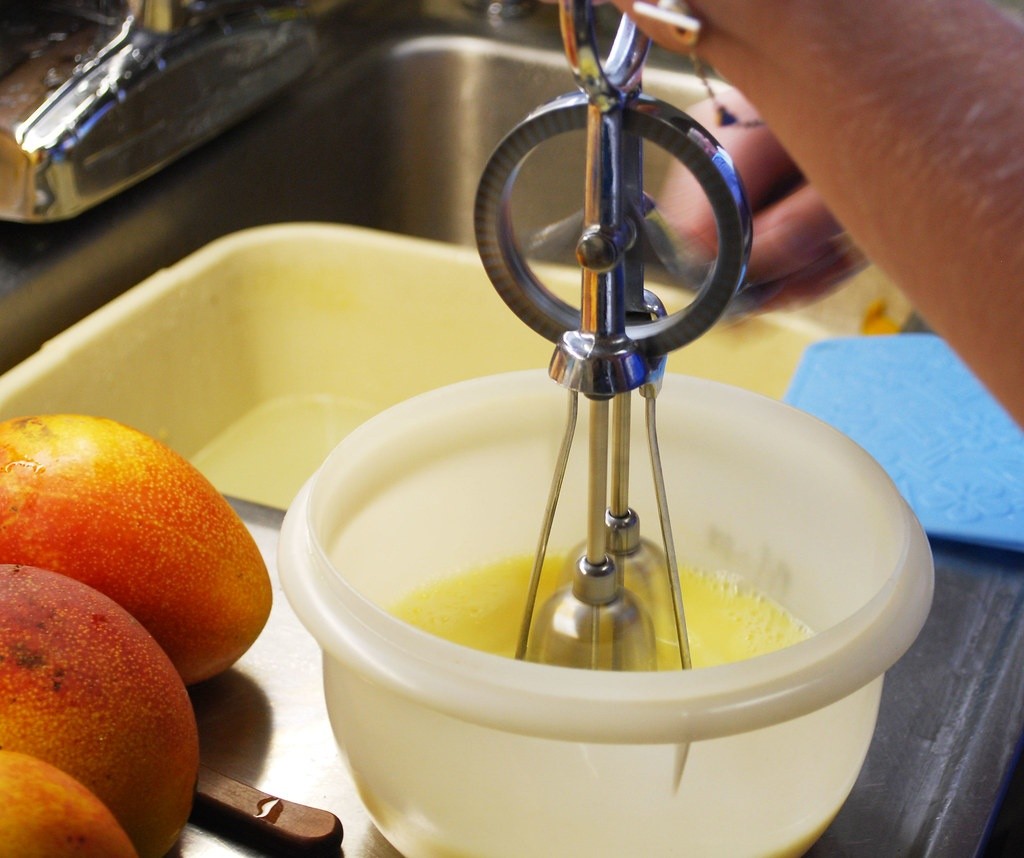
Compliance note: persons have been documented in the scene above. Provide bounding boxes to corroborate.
[610,0,1024,437]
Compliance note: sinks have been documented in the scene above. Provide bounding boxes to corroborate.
[0,17,930,531]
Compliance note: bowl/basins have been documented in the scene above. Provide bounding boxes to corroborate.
[278,371,935,858]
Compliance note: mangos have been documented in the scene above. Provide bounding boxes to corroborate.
[0,414,274,858]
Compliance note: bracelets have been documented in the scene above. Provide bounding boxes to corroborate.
[630,1,765,129]
